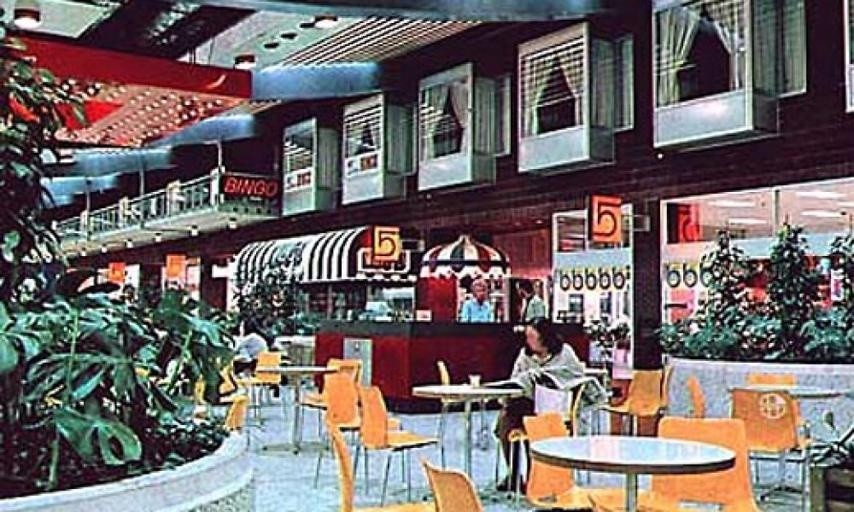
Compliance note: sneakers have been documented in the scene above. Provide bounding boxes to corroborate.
[495,475,525,493]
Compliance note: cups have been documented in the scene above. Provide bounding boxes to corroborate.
[468,375,480,388]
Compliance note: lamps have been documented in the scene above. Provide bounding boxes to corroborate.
[78,215,242,259]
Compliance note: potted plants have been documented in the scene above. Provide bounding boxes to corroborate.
[639,216,854,497]
[0,0,260,512]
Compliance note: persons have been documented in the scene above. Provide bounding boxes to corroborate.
[457,279,495,324]
[496,316,586,491]
[515,278,544,321]
[120,283,137,300]
[230,319,268,374]
